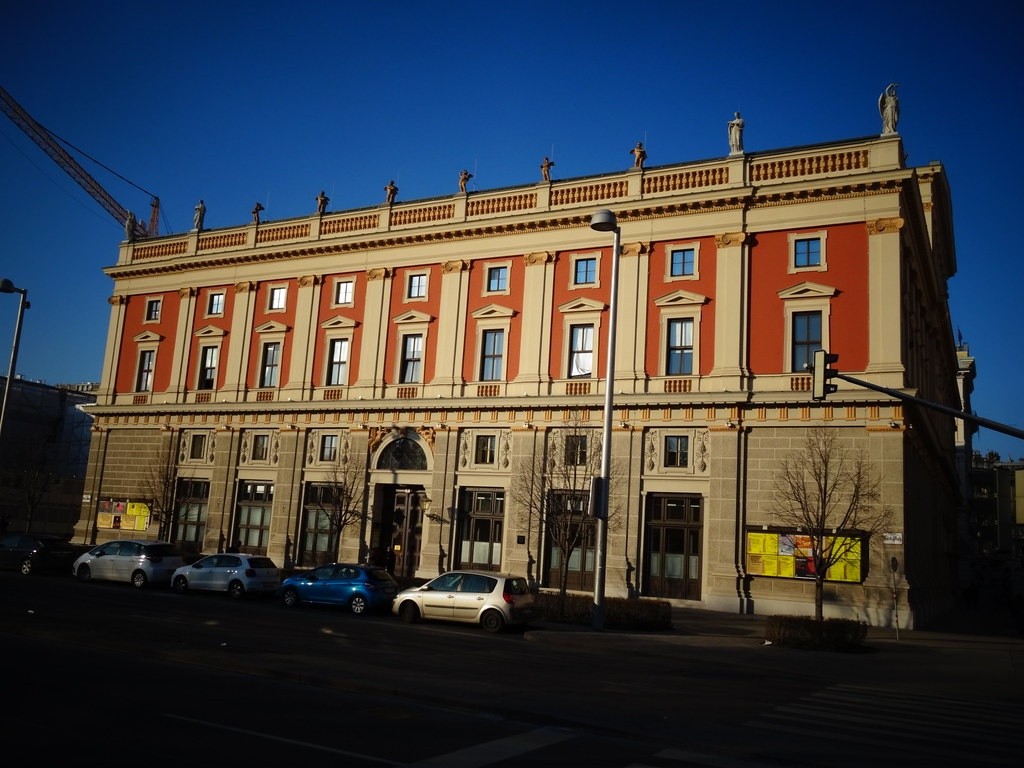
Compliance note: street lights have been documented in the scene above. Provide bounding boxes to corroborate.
[587,209,624,636]
[0,278,30,442]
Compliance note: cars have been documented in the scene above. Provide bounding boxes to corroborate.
[72,540,184,591]
[171,552,280,598]
[280,562,400,617]
[392,569,535,634]
[1,535,76,577]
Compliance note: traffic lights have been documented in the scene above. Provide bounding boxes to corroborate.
[810,350,841,406]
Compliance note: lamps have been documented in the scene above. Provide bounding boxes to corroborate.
[420,493,445,524]
[341,489,368,517]
